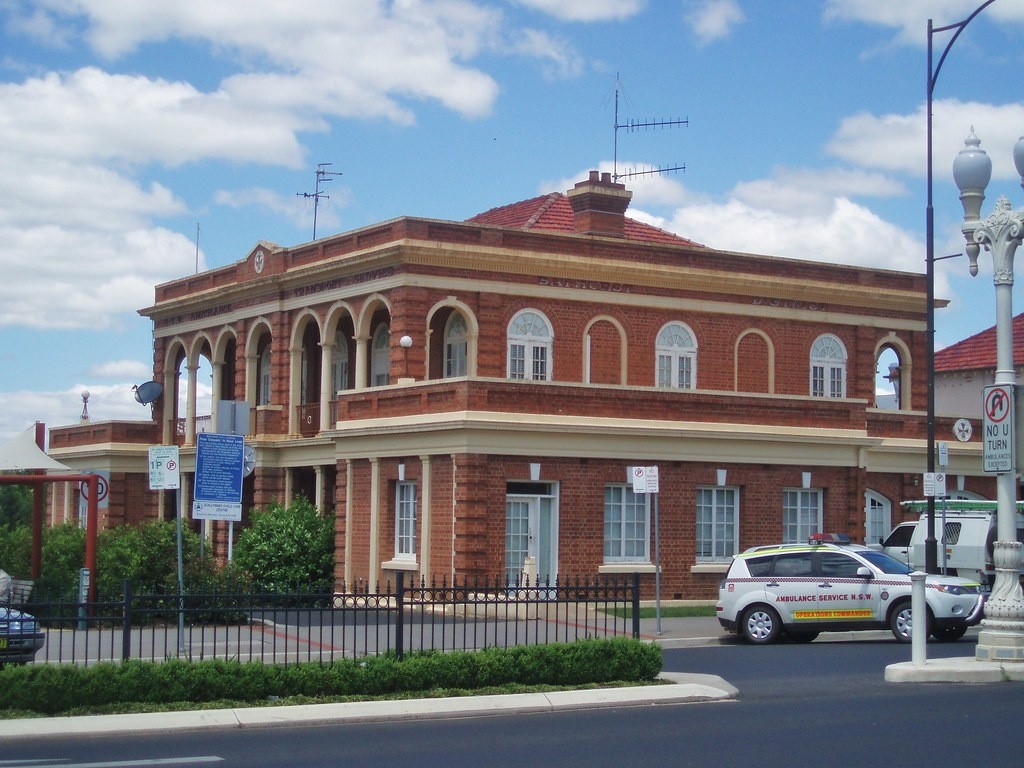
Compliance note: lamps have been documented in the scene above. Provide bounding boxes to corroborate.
[397,336,416,386]
[77,391,92,425]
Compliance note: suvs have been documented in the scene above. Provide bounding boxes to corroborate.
[716,543,986,645]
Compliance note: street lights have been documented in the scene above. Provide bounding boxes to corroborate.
[953,126,1024,665]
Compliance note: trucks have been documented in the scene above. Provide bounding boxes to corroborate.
[878,500,1024,597]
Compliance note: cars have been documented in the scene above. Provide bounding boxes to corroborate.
[0,607,45,668]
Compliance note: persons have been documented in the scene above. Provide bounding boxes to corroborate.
[0,569,14,606]
